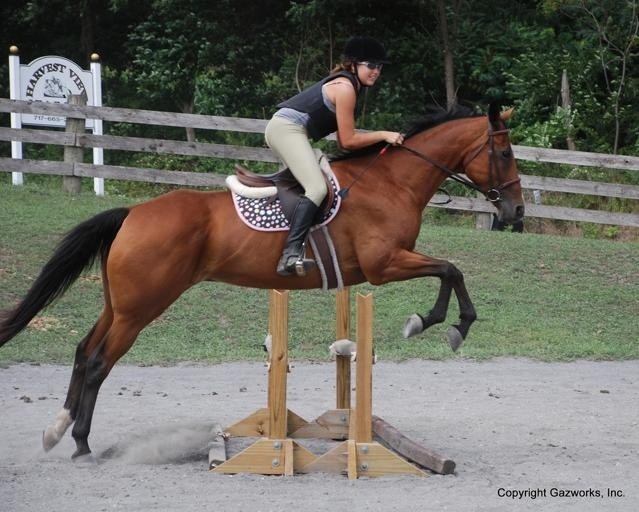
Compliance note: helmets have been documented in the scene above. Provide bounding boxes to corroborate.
[342,37,386,62]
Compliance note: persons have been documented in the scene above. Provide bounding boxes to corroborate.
[264,36,406,278]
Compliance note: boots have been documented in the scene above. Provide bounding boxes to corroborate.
[276,194,317,277]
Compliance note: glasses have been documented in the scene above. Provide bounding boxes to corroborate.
[357,61,385,71]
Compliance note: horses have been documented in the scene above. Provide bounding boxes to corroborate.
[0,96,526,468]
